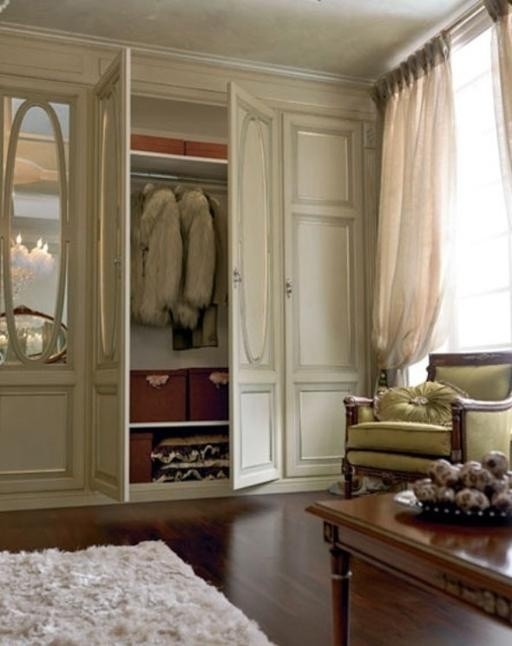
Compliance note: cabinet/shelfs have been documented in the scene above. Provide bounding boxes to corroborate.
[84,48,382,504]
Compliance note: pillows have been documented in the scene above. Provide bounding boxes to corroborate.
[373,383,470,427]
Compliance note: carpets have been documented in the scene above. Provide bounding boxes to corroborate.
[0,538,276,646]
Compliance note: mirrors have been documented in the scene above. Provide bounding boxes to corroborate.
[0,85,81,384]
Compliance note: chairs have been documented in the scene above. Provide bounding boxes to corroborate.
[342,352,511,498]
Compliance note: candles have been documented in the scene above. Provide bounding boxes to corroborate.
[35,237,42,250]
[43,240,49,253]
[16,231,22,244]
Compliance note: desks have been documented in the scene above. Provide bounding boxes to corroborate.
[305,490,511,645]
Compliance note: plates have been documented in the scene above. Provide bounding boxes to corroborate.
[394,489,510,524]
